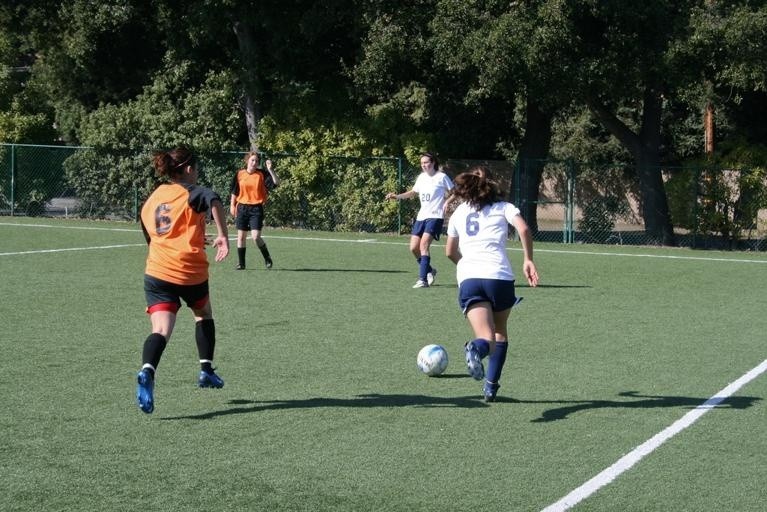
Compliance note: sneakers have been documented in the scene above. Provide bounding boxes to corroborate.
[265,257,272,268]
[136,368,154,414]
[427,269,436,285]
[481,386,496,402]
[464,341,483,380]
[413,280,429,289]
[199,369,224,389]
[236,263,245,269]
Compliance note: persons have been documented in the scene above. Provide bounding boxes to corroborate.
[448,165,539,402]
[230,150,279,271]
[385,152,457,288]
[135,146,230,413]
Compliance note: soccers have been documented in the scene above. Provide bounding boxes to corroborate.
[417,343,448,376]
